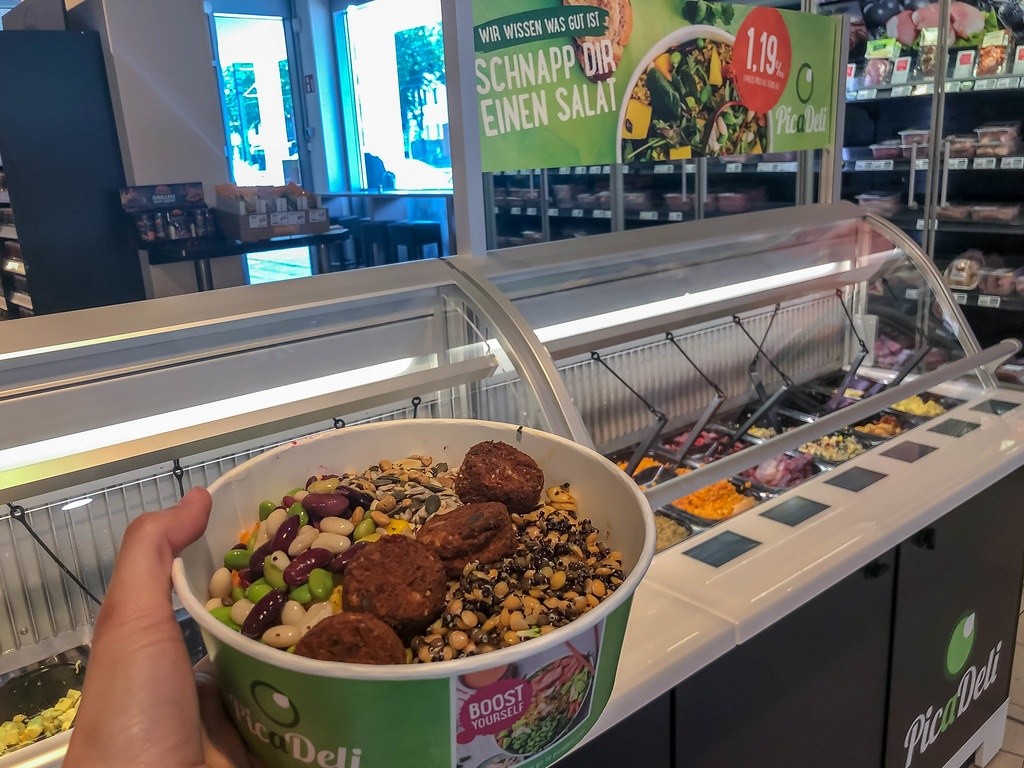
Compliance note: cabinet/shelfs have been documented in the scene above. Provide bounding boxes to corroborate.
[1,150,38,322]
[2,198,1023,768]
[483,33,1023,374]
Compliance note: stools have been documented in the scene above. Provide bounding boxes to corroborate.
[327,212,443,273]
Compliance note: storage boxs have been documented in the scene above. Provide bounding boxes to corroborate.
[207,182,330,242]
